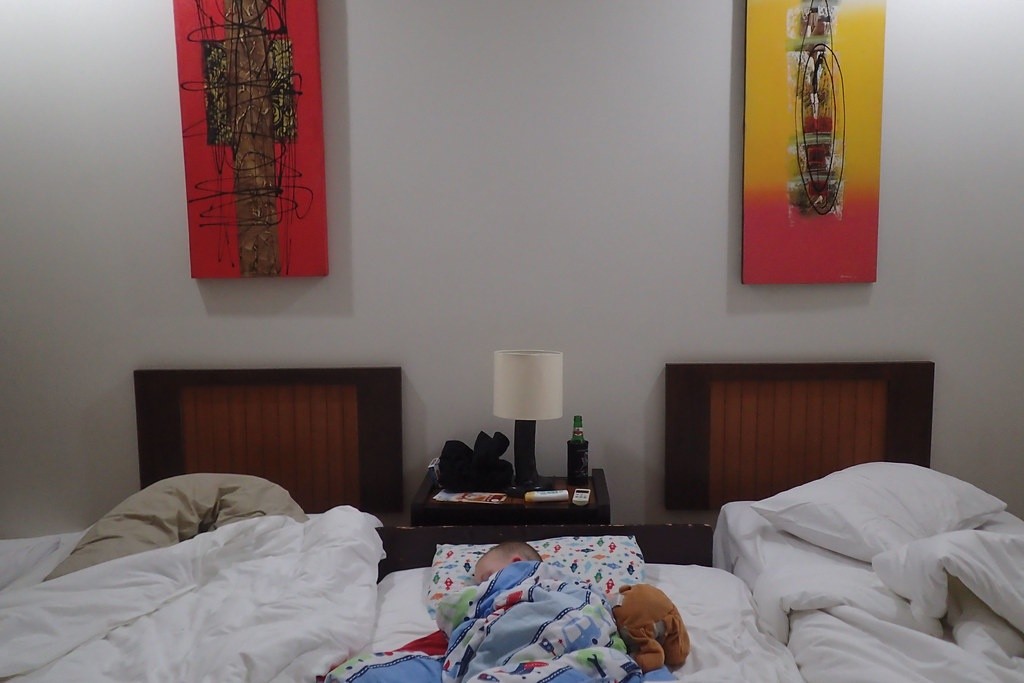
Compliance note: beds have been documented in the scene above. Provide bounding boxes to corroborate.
[0,360,935,682]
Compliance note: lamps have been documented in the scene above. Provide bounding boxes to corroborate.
[492,349,564,499]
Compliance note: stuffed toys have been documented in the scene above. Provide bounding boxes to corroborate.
[613,584,689,671]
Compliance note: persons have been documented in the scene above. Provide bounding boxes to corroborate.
[475,541,611,683]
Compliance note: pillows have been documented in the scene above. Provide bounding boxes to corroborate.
[427,535,645,621]
[748,462,1008,563]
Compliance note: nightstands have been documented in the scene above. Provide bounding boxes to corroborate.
[410,460,611,525]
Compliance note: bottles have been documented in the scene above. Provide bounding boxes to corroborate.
[567,416,588,485]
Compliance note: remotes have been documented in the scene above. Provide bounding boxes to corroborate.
[525,490,569,502]
[573,489,591,506]
